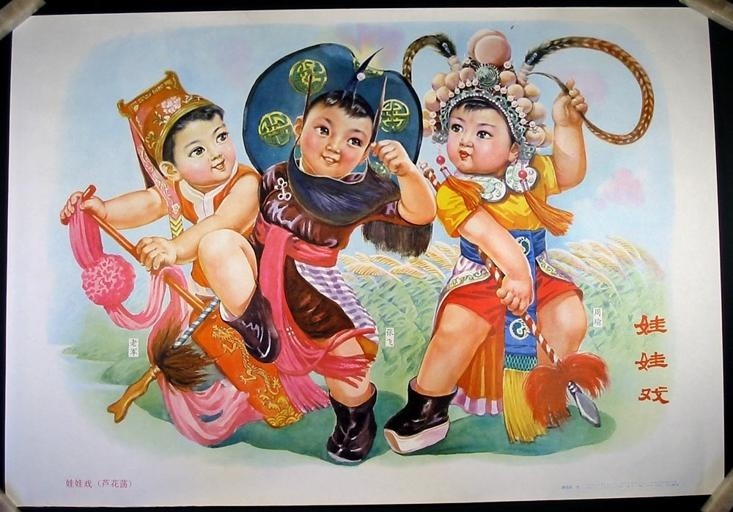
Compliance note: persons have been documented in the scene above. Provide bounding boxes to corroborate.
[198,90,436,465]
[60,103,262,326]
[383,79,588,454]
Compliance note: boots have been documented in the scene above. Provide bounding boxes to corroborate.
[219,280,280,364]
[382,377,458,454]
[325,380,377,463]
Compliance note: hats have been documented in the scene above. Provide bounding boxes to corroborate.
[117,72,214,171]
